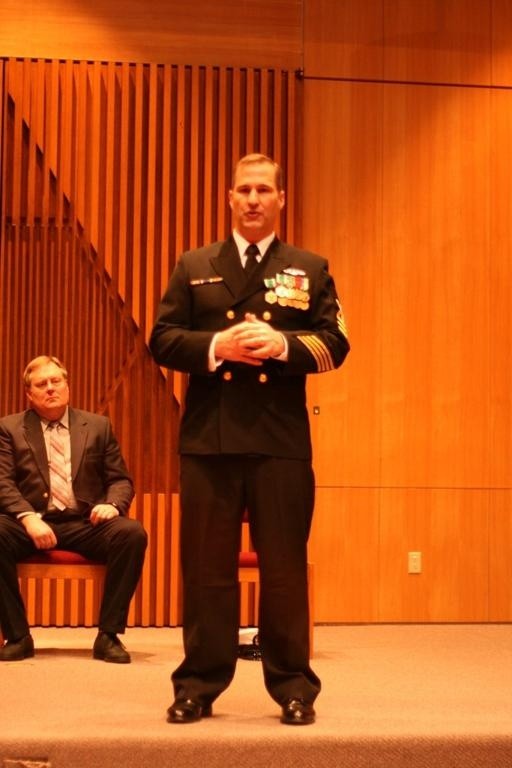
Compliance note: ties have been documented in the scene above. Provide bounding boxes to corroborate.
[244,243,260,276]
[48,420,71,512]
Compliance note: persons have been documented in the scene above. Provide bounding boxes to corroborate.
[149,152,352,728]
[1,353,149,665]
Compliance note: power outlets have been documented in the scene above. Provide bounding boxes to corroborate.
[408,550,422,573]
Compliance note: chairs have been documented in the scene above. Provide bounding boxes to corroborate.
[0,546,106,647]
[234,507,315,659]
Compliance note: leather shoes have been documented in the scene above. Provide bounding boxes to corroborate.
[0,633,35,661]
[164,698,212,721]
[282,696,315,726]
[93,632,132,663]
